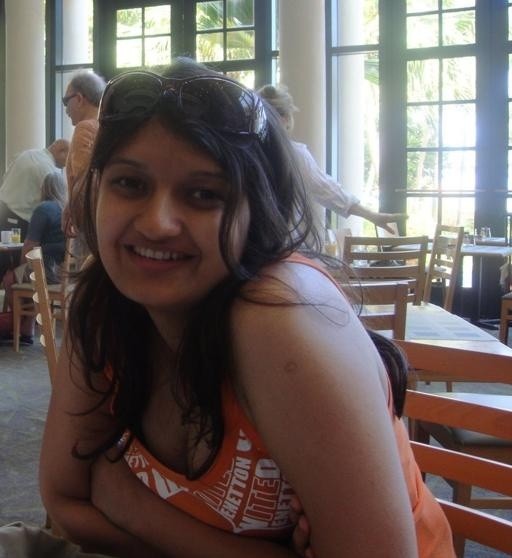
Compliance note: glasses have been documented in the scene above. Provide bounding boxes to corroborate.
[61,95,77,106]
[96,71,267,144]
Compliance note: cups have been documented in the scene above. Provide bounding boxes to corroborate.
[472,225,492,241]
[0,227,20,244]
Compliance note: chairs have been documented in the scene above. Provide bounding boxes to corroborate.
[11,222,512,558]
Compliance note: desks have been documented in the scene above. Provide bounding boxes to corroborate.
[0,242,24,269]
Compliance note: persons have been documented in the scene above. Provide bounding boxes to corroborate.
[1,55,460,556]
[0,138,72,289]
[1,171,71,344]
[61,68,110,326]
[255,83,410,268]
